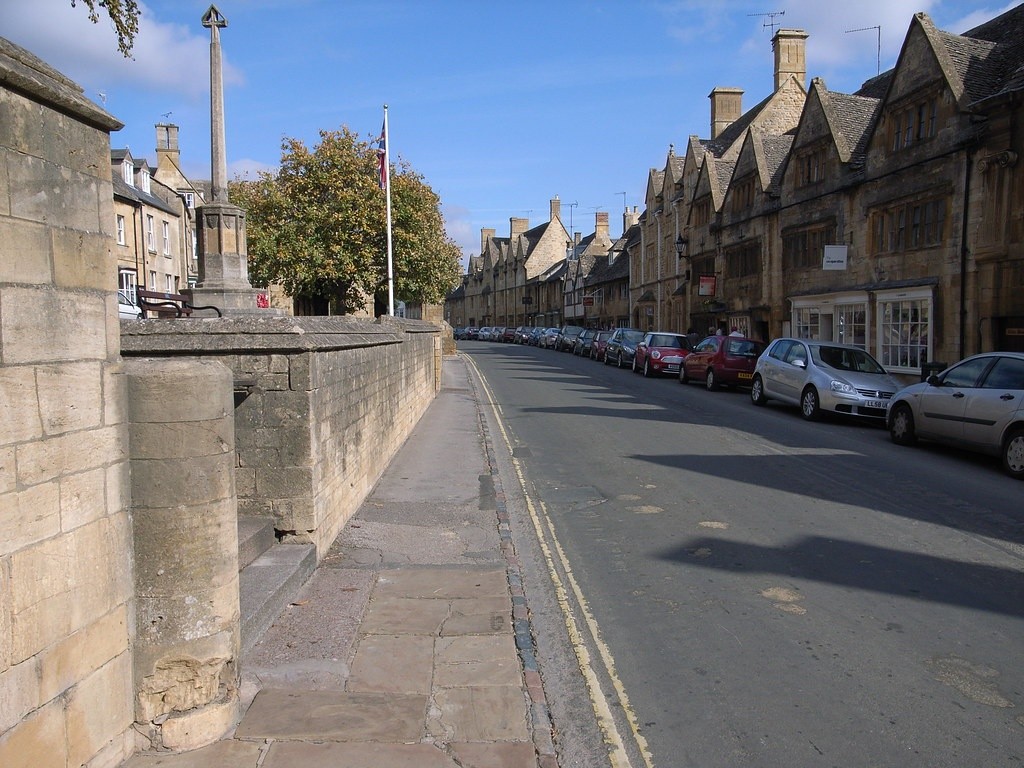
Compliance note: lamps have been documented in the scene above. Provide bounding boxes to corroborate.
[674,232,690,261]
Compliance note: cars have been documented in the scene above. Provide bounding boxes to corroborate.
[555,326,584,353]
[574,330,598,357]
[453,326,517,343]
[590,331,614,361]
[118,291,143,320]
[632,332,693,378]
[513,326,561,349]
[604,330,645,368]
[751,338,908,419]
[679,336,769,391]
[887,352,1024,479]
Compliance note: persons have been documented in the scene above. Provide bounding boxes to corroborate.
[645,321,657,345]
[588,323,615,331]
[560,322,563,329]
[635,321,639,328]
[565,319,576,326]
[706,326,745,352]
[687,327,700,345]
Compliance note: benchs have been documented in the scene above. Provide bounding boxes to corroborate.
[136,284,222,320]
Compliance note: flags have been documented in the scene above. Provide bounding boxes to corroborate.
[377,119,387,190]
